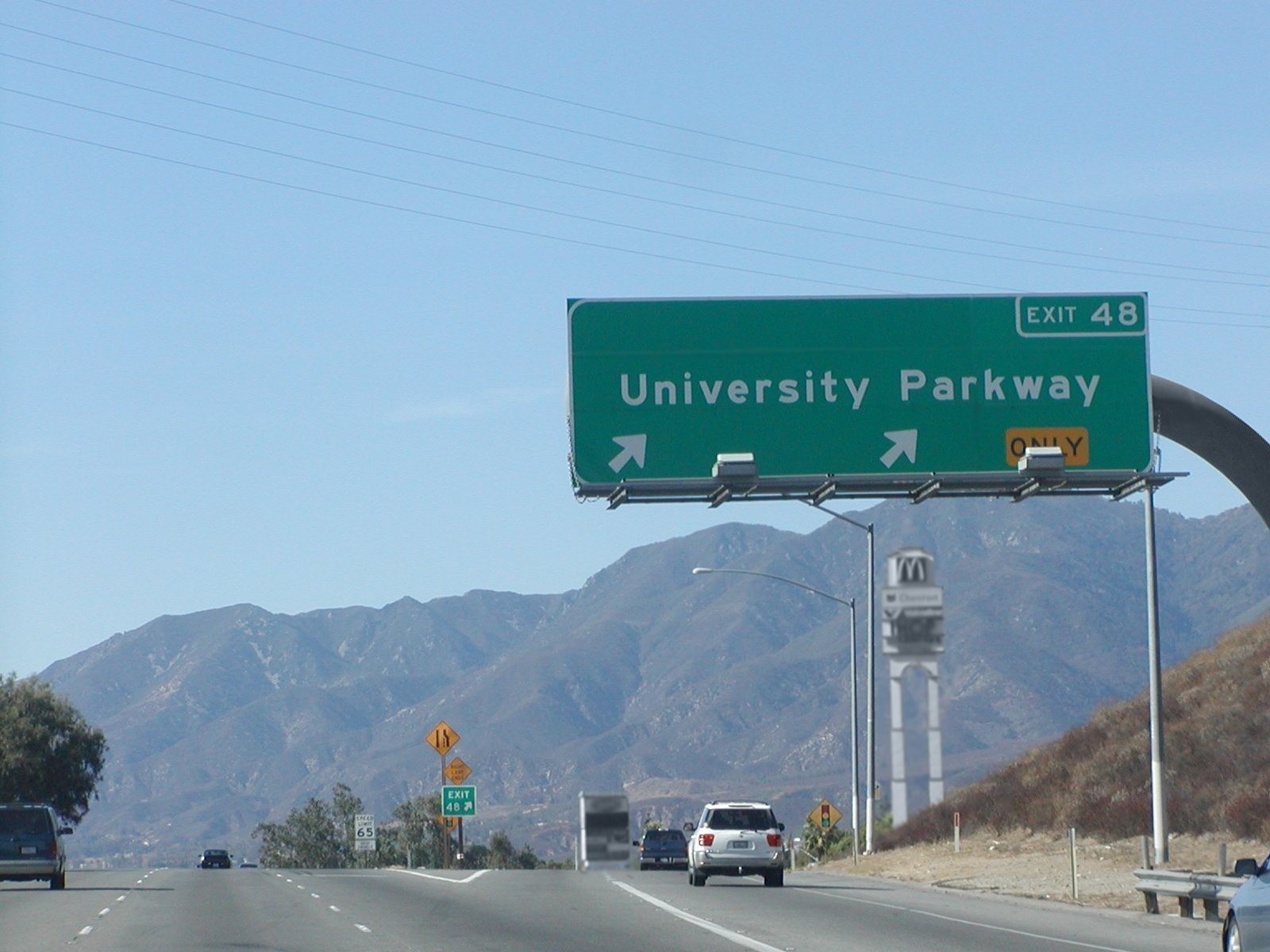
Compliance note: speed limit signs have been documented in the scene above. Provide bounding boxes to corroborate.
[355,814,376,840]
[442,785,478,817]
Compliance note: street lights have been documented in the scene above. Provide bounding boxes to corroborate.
[692,567,861,855]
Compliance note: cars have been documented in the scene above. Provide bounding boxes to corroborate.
[1222,853,1270,951]
[198,849,234,869]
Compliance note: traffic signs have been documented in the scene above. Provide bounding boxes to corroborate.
[565,297,1154,488]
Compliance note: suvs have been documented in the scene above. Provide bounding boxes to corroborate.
[633,830,689,871]
[683,799,785,887]
[0,803,73,889]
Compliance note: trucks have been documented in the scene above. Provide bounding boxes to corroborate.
[577,791,631,871]
